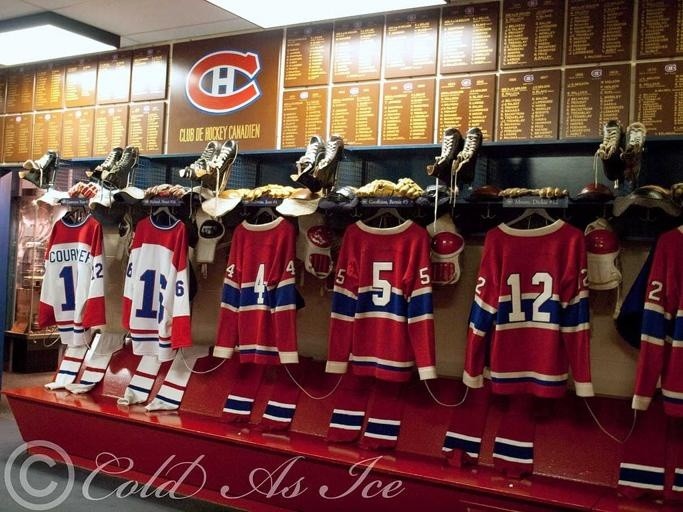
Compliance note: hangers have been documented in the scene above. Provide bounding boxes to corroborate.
[52,205,102,231]
[232,207,297,234]
[344,207,428,240]
[485,208,585,242]
[135,206,186,233]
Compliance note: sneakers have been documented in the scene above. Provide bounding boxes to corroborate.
[101,147,138,190]
[19,150,58,189]
[427,128,463,187]
[290,136,323,193]
[90,148,123,183]
[180,141,220,188]
[599,120,625,181]
[196,140,238,197]
[314,136,344,191]
[452,128,482,186]
[621,123,646,180]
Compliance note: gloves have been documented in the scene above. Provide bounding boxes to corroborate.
[395,178,423,199]
[145,184,171,200]
[530,186,568,199]
[68,182,83,199]
[355,180,395,197]
[223,185,292,200]
[82,183,98,200]
[498,188,531,197]
[169,183,185,199]
